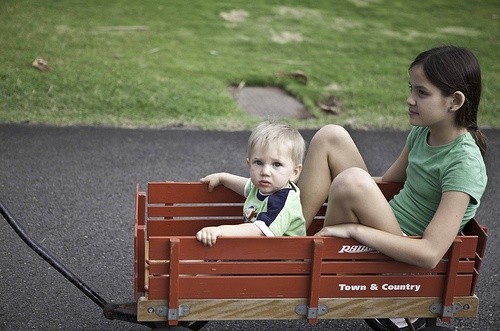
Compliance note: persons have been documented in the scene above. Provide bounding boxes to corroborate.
[242,41,489,280]
[193,114,308,281]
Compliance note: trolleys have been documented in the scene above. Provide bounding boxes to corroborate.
[1,182,488,331]
